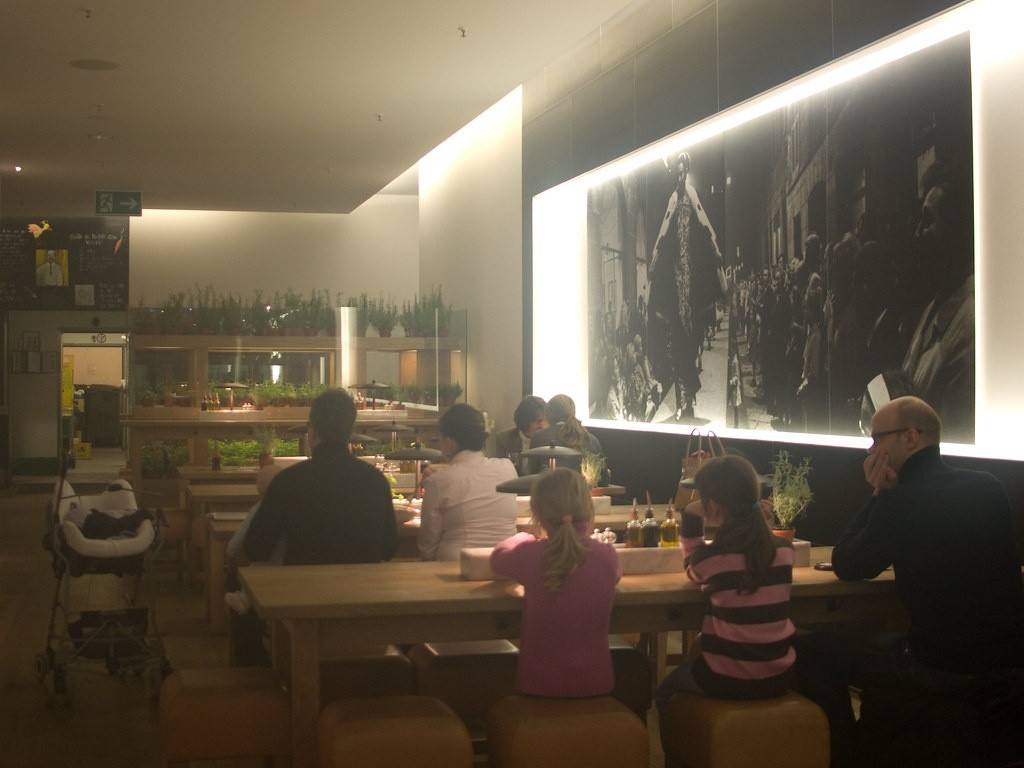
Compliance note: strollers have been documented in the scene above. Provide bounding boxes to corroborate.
[36,443,173,695]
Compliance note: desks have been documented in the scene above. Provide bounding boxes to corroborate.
[181,480,901,768]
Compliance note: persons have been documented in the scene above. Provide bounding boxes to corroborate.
[495,467,623,699]
[529,395,609,486]
[653,453,797,703]
[595,151,975,442]
[223,387,398,666]
[494,394,550,476]
[416,404,519,561]
[36,249,64,287]
[799,396,1024,768]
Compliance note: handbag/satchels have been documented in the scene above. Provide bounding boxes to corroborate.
[674,428,726,509]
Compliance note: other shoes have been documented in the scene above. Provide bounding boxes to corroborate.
[235,646,268,667]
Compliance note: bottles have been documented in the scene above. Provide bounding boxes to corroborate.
[201,393,221,410]
[625,506,681,549]
[590,527,617,545]
[211,448,221,472]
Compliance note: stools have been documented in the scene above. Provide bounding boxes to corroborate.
[160,633,1024,768]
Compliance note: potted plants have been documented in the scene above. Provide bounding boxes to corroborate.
[130,370,463,412]
[124,423,442,472]
[581,451,607,496]
[128,285,451,338]
[770,449,815,544]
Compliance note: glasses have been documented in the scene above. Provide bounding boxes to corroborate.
[871,428,922,444]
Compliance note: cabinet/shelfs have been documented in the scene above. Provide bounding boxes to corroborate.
[124,334,471,571]
